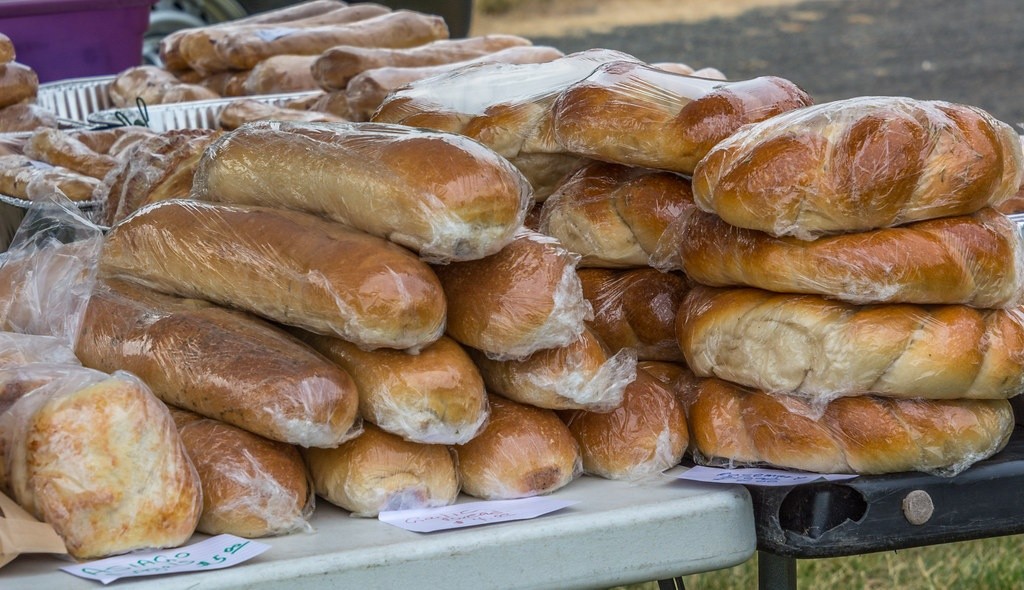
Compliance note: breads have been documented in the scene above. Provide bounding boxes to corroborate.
[0,0,1024,559]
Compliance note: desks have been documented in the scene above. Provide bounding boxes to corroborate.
[0,465,757,590]
[747,426,1024,590]
